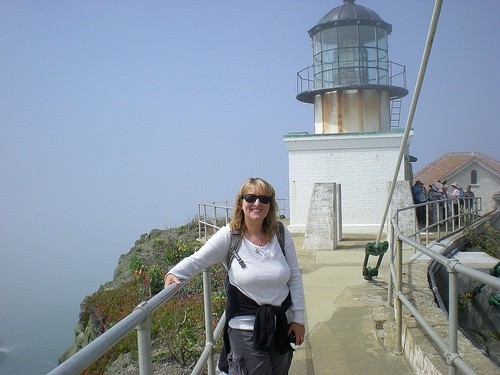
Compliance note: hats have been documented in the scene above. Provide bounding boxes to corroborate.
[429,182,440,192]
[451,182,459,189]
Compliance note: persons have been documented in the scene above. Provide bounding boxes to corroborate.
[413,180,475,226]
[164,177,305,375]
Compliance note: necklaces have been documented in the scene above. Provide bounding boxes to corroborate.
[246,231,263,252]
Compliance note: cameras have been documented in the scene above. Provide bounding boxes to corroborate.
[289,331,296,343]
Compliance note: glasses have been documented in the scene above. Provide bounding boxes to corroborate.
[242,194,272,204]
